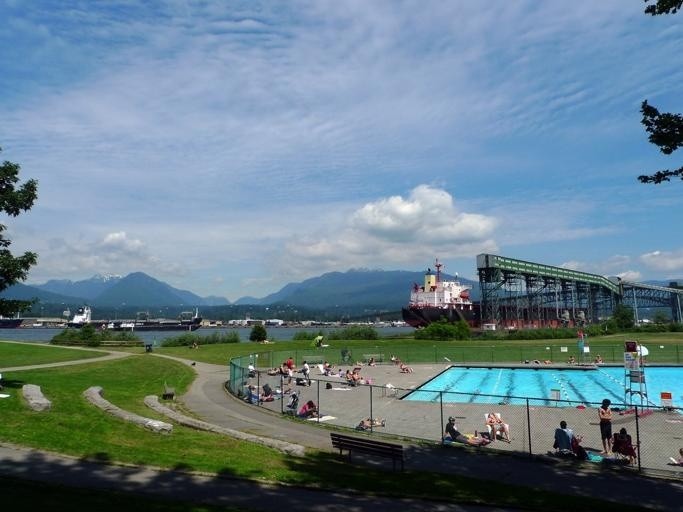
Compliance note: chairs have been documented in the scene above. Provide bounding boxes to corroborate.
[484,413,509,440]
[555,428,637,468]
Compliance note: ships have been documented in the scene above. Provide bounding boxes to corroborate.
[65,305,202,330]
[403,259,587,329]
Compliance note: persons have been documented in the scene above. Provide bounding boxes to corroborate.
[567,355,575,364]
[439,399,683,466]
[241,354,411,429]
[594,355,602,363]
